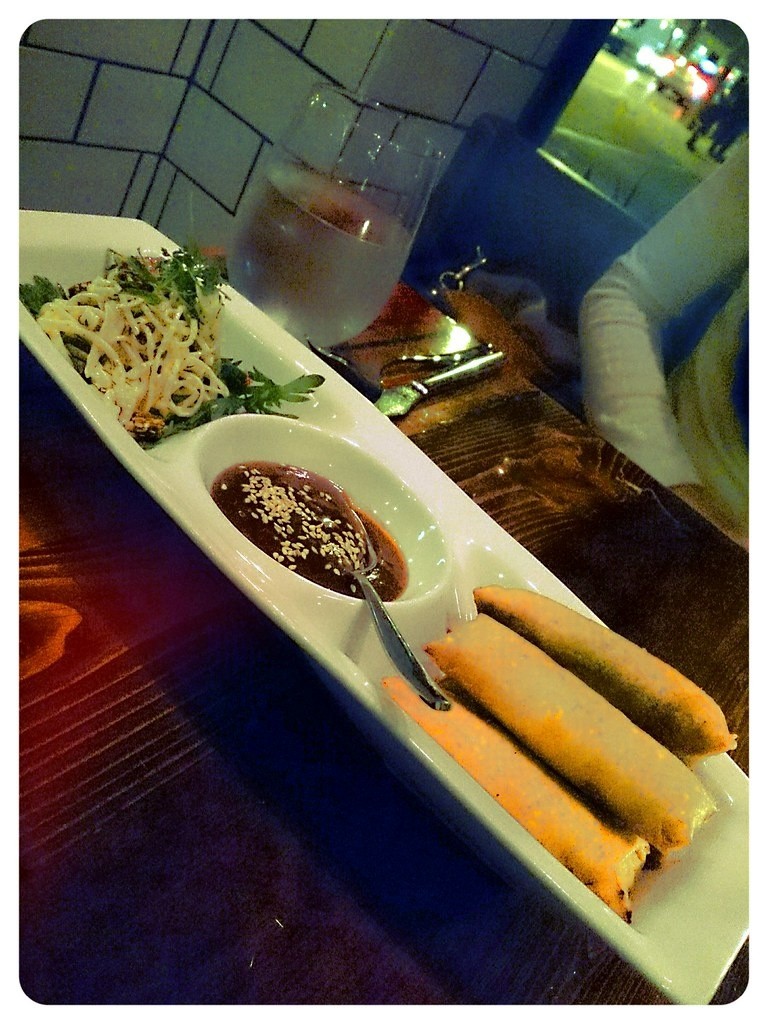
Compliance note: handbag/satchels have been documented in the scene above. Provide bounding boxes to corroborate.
[442,249,578,380]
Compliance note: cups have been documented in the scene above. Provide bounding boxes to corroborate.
[216,82,444,359]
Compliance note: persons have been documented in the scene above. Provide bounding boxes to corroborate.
[687,75,749,163]
[580,139,749,556]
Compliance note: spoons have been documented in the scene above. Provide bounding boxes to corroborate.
[348,505,450,712]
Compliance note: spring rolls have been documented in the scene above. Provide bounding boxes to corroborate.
[380,586,737,922]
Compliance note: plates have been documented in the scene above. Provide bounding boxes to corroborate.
[21,207,747,1007]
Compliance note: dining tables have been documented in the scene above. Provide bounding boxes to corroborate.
[19,242,750,1007]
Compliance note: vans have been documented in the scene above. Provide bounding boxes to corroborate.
[607,19,679,58]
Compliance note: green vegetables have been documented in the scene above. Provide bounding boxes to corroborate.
[19,241,327,450]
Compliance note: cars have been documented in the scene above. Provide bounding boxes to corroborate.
[661,60,715,104]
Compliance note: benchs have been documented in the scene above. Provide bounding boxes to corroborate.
[397,116,749,456]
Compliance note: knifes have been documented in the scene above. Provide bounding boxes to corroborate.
[373,352,505,416]
